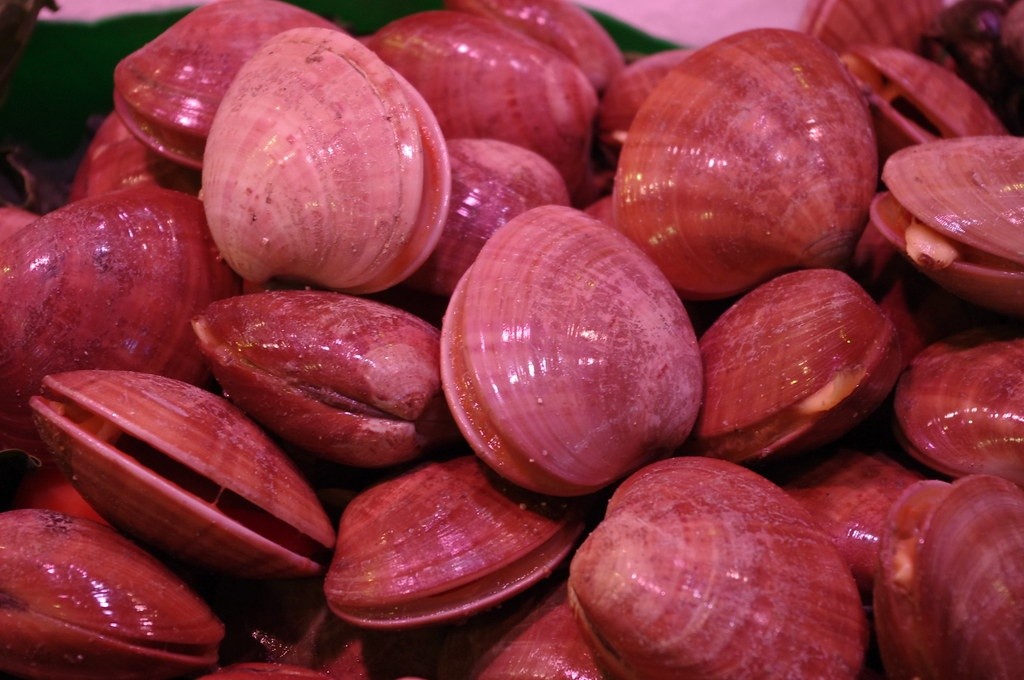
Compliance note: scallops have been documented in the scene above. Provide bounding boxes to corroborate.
[0,1,1024,680]
